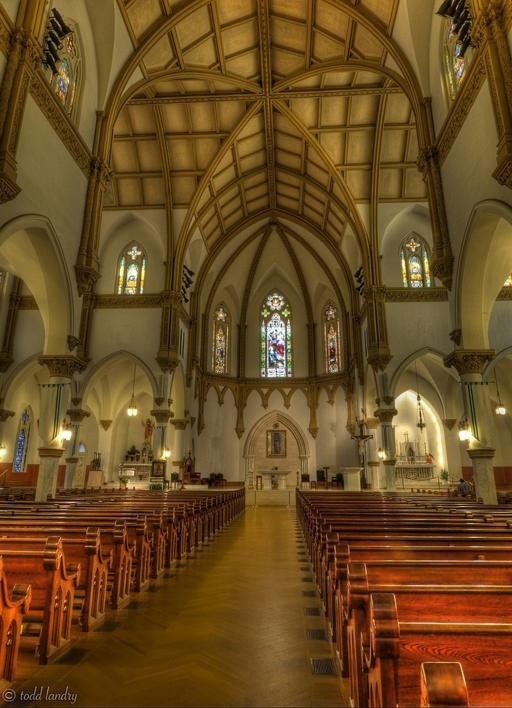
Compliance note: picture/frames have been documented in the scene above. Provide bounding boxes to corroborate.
[151,461,165,476]
[264,429,288,457]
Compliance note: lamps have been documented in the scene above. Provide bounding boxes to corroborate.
[414,358,427,431]
[455,383,471,443]
[492,366,507,415]
[126,361,139,416]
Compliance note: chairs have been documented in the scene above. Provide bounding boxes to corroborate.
[302,469,344,489]
[191,472,227,488]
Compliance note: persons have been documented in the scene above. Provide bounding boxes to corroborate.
[457,478,474,497]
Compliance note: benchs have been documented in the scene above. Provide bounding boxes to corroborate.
[1,581,74,681]
[1,487,246,537]
[1,538,202,583]
[72,580,152,631]
